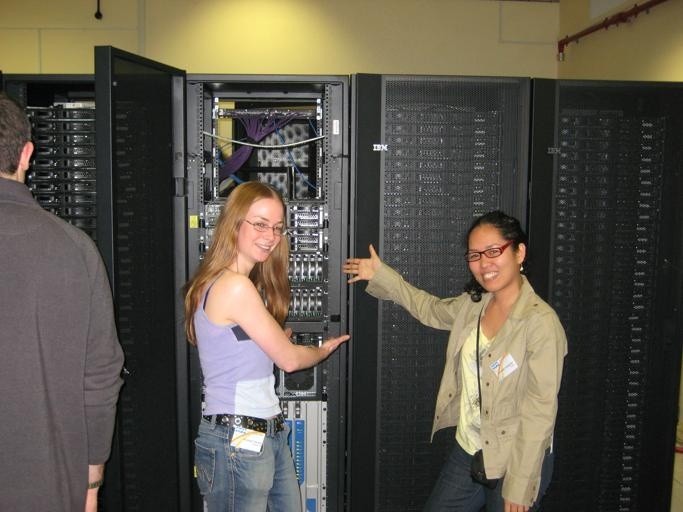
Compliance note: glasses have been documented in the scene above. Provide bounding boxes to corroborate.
[244,219,284,236]
[464,240,514,262]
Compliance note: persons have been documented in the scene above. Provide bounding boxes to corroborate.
[340,208,569,512]
[175,180,353,512]
[0,97,127,512]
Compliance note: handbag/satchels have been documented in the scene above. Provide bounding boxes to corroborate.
[469,447,500,490]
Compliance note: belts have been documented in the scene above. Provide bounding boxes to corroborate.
[202,411,285,433]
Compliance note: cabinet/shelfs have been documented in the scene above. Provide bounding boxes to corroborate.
[2,71,182,511]
[173,70,372,510]
[534,77,681,510]
[379,75,531,509]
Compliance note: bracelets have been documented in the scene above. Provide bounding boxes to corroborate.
[87,480,104,491]
